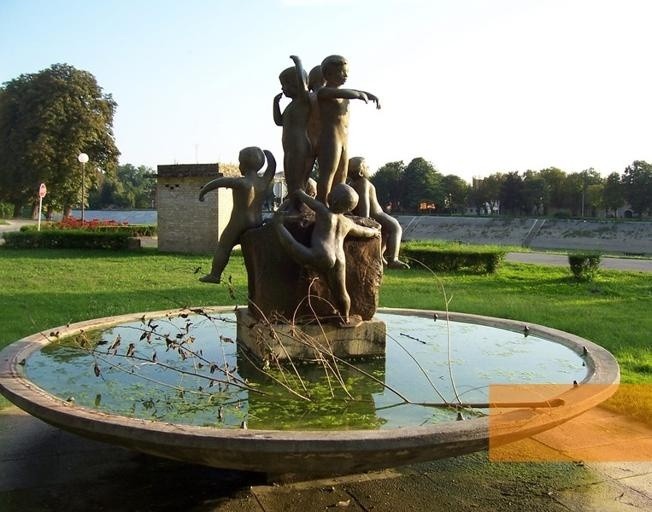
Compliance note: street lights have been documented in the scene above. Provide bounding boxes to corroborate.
[78,152,90,226]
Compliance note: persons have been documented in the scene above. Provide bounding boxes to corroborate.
[315,55,381,209]
[271,55,320,199]
[346,155,411,271]
[275,182,382,322]
[198,145,277,284]
[304,65,331,163]
